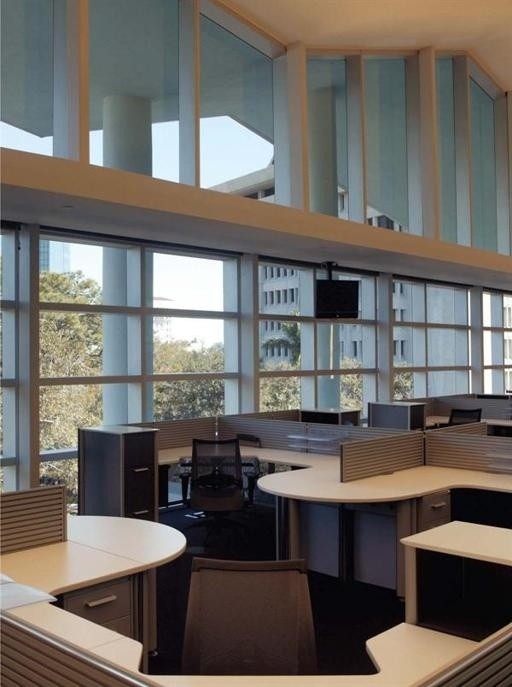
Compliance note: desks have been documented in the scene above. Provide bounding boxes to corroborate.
[77,394,512,541]
[0,514,512,687]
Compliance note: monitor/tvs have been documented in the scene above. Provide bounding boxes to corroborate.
[315,280,359,319]
